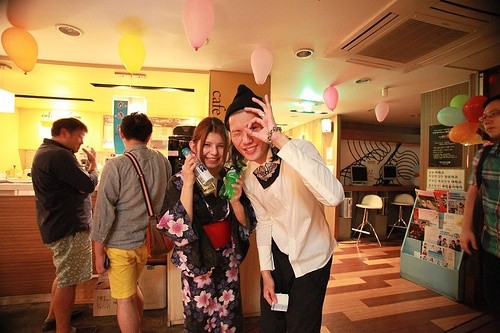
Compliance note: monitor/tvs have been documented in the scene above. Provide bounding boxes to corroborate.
[351,166,368,183]
[383,165,398,184]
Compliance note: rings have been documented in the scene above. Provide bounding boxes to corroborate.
[261,113,265,118]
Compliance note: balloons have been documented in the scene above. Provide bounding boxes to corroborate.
[251,48,273,85]
[375,103,389,122]
[1,26,38,74]
[183,0,215,51]
[323,86,338,110]
[438,94,488,143]
[118,34,146,74]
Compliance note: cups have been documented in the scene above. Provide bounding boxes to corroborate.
[78,146,92,165]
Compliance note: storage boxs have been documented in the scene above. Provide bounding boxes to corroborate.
[92,264,166,316]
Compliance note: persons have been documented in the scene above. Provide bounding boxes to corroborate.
[224,85,345,333]
[158,117,257,333]
[458,93,500,320]
[437,236,461,252]
[89,112,172,333]
[30,118,98,333]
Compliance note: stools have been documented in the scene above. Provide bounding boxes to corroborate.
[387,194,414,238]
[351,194,383,247]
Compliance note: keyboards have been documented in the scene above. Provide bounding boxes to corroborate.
[351,184,372,186]
[383,184,402,186]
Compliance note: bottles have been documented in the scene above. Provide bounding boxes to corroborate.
[219,160,244,200]
[194,175,216,195]
[182,147,215,185]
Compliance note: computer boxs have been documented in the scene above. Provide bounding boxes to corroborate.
[378,197,389,216]
[339,198,353,218]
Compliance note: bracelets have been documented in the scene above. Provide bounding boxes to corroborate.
[268,126,282,148]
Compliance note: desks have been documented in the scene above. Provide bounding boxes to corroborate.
[336,185,418,241]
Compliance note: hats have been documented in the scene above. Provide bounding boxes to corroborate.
[225,84,265,131]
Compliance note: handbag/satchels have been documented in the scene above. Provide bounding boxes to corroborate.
[148,215,175,258]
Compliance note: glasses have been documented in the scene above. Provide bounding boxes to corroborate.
[478,111,499,122]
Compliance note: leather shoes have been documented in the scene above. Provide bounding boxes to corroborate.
[42,309,84,331]
